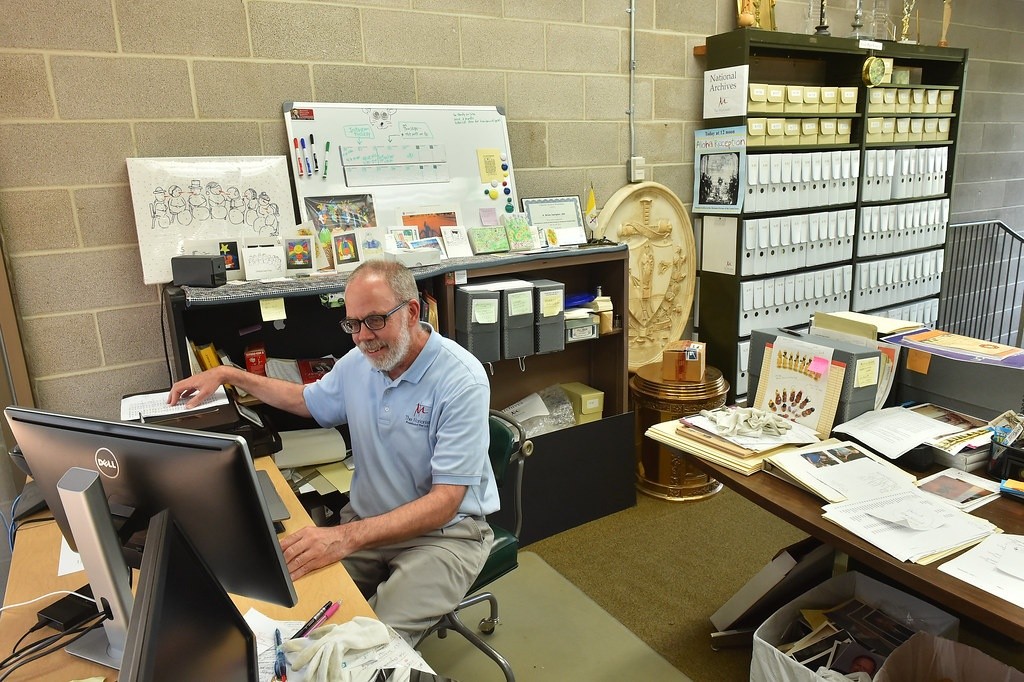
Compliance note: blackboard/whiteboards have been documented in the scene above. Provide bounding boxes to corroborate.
[282,102,520,233]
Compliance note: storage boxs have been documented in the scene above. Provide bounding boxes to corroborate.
[936,91,954,114]
[454,147,1024,682]
[746,118,767,146]
[766,118,785,145]
[909,89,925,113]
[837,87,858,113]
[896,89,911,112]
[747,83,768,112]
[835,119,852,143]
[803,86,820,113]
[883,88,897,112]
[800,118,819,144]
[784,86,804,113]
[819,87,838,113]
[866,117,951,143]
[817,119,837,144]
[766,84,786,112]
[868,88,884,113]
[784,119,801,145]
[923,89,939,113]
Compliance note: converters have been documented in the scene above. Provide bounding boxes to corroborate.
[37,583,99,632]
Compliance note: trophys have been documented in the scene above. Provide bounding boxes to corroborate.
[851,0,921,44]
[805,0,831,37]
[937,0,952,47]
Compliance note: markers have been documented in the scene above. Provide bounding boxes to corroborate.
[322,141,330,178]
[300,137,312,177]
[293,138,304,176]
[309,134,319,172]
[296,271,338,278]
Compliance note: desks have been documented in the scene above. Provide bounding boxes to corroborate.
[642,311,1024,644]
[0,456,439,682]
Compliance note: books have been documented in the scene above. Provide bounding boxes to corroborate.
[185,336,246,399]
[933,443,991,474]
[646,409,799,476]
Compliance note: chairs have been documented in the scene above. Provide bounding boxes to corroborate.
[375,409,533,682]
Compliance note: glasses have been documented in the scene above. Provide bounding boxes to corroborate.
[339,299,410,334]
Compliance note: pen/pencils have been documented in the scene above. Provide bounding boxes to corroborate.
[289,601,333,640]
[137,412,145,425]
[303,598,344,638]
[274,627,288,682]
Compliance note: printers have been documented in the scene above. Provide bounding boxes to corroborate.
[122,383,284,460]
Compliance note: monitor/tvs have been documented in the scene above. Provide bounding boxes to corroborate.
[6,404,298,682]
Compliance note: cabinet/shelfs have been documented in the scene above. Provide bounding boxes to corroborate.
[692,27,970,410]
[154,237,638,552]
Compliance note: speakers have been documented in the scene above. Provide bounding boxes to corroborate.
[171,256,227,288]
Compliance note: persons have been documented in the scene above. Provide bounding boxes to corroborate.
[168,258,498,682]
[832,654,876,681]
